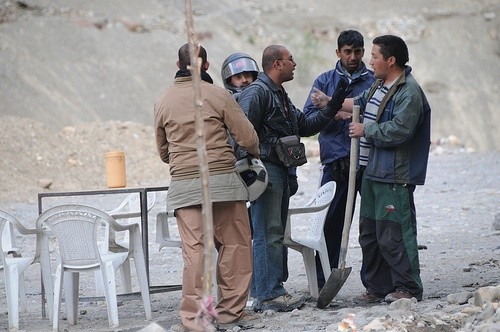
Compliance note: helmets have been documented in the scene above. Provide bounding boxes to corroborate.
[220,52,261,92]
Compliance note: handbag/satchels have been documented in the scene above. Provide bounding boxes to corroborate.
[276,135,308,168]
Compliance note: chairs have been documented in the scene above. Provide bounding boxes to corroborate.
[93,190,156,308]
[282,180,337,297]
[157,210,183,254]
[0,209,54,332]
[35,203,153,332]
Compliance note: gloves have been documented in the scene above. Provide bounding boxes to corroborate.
[326,75,355,112]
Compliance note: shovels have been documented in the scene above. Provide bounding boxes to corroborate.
[317,105,361,309]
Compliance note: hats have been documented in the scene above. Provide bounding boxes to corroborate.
[234,157,270,202]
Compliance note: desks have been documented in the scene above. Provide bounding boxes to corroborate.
[37,186,169,321]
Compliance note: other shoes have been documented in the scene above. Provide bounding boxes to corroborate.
[251,292,306,313]
[352,291,383,305]
[216,318,255,332]
[384,290,414,304]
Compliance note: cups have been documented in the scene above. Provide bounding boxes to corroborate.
[104,151,126,188]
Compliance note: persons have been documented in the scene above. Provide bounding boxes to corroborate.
[154,41,262,332]
[226,30,431,310]
